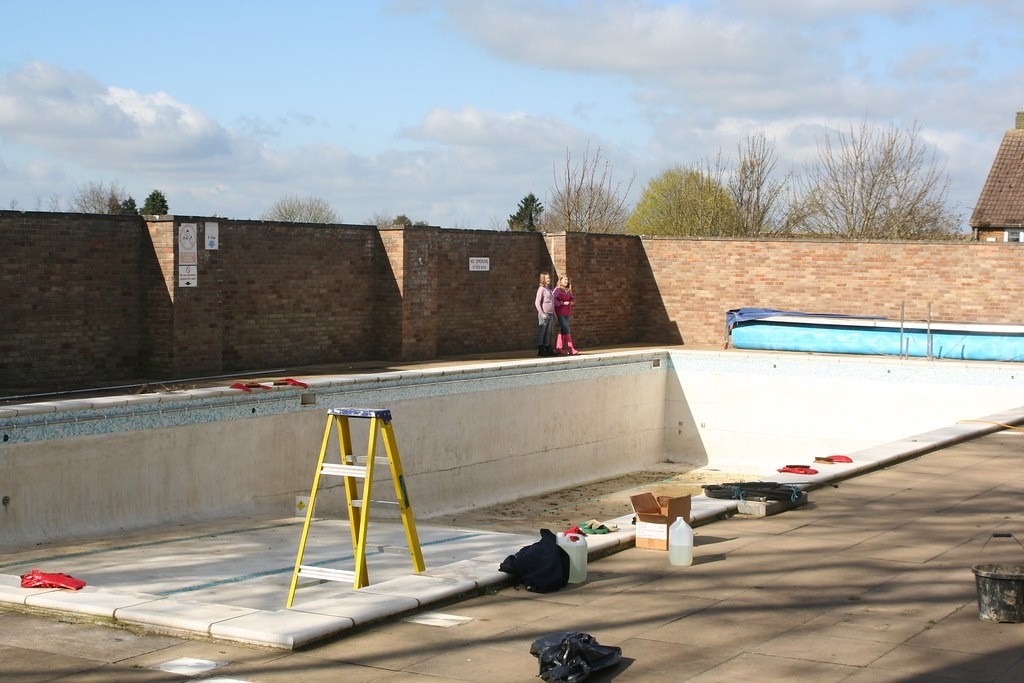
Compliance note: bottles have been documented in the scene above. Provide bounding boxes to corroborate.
[669,517,693,566]
[556,532,587,583]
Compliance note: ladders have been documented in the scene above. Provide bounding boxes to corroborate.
[284,407,426,610]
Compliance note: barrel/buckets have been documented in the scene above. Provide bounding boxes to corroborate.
[972,534,1024,624]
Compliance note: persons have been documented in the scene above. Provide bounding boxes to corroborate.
[551,273,578,355]
[533,271,557,356]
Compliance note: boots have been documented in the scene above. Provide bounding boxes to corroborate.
[565,334,578,355]
[555,333,567,355]
[537,345,549,356]
[545,345,556,356]
